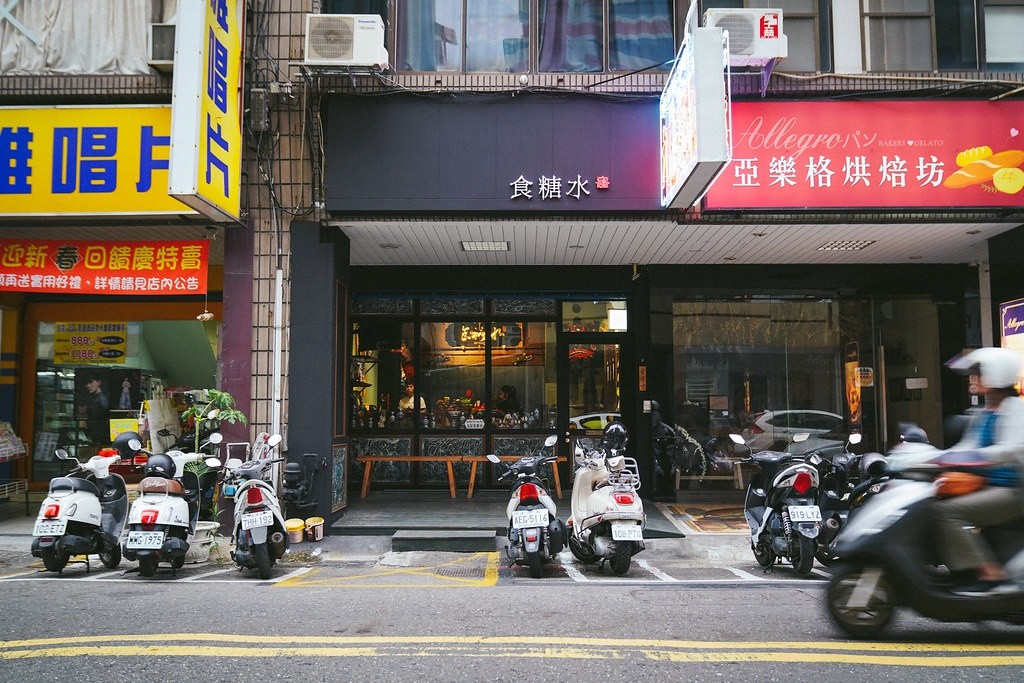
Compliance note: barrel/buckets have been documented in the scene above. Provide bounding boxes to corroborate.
[284,518,305,544]
[305,517,325,542]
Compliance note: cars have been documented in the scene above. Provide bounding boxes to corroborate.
[733,410,844,453]
[569,413,621,429]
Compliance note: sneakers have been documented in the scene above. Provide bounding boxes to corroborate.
[948,575,1021,597]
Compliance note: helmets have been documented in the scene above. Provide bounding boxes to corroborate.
[112,431,144,459]
[144,453,176,479]
[948,347,1020,389]
[860,452,891,479]
[601,420,629,450]
[903,427,930,444]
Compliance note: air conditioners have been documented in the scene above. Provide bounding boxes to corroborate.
[302,12,387,71]
[703,7,789,67]
[147,23,177,72]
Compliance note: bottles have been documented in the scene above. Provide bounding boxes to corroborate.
[351,405,465,428]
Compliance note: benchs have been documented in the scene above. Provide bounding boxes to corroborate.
[356,454,462,499]
[463,455,567,499]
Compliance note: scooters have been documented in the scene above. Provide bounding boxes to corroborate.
[805,430,866,567]
[566,421,647,575]
[824,450,1024,637]
[157,427,222,455]
[205,433,290,579]
[113,431,226,576]
[30,449,129,575]
[729,431,822,573]
[485,435,568,579]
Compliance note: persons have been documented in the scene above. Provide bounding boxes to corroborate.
[893,347,1024,590]
[77,370,109,443]
[398,382,426,412]
[496,385,521,410]
[119,376,132,409]
